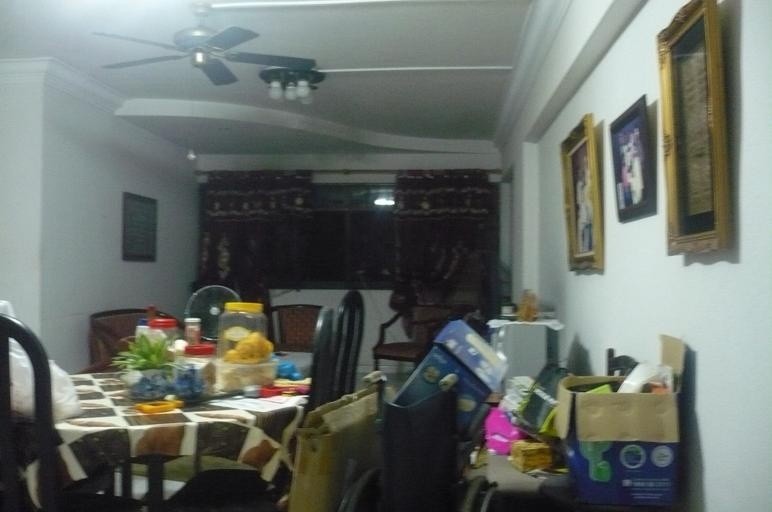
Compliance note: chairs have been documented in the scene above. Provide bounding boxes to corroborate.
[456,347,642,511]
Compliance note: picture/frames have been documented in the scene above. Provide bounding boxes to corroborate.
[656,0,729,255]
[121,193,159,261]
[608,95,655,222]
[559,113,603,271]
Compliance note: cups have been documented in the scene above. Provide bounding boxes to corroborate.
[166,360,208,403]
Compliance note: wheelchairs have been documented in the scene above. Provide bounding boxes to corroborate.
[336,367,510,510]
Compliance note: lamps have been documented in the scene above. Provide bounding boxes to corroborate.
[374,188,395,205]
[258,66,325,104]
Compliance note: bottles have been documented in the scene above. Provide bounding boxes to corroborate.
[215,302,266,366]
[136,318,181,356]
[183,315,202,346]
[519,288,540,323]
[501,296,516,315]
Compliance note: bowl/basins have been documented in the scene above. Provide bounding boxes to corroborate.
[121,373,166,400]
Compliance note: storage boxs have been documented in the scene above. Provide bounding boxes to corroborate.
[287,384,392,512]
[432,319,508,393]
[394,341,492,440]
[554,336,686,506]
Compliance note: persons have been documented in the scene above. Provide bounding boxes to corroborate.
[621,127,645,207]
[0,300,84,421]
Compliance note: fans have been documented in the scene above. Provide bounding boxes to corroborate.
[91,1,317,85]
